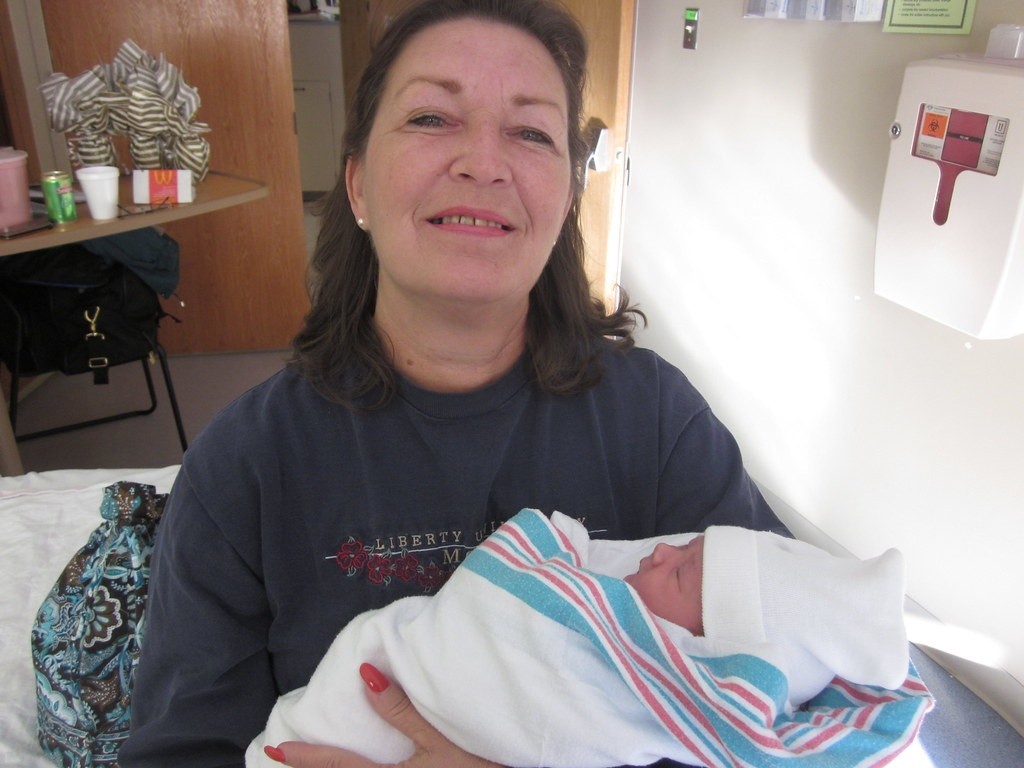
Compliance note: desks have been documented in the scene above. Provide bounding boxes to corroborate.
[1,171,270,476]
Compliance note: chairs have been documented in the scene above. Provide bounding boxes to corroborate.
[0,298,187,453]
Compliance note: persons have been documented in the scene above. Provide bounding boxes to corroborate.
[245,508,910,768]
[118,0,798,768]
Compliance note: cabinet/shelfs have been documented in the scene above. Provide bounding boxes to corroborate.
[292,78,338,192]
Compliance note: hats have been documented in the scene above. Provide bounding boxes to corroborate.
[699,516,911,717]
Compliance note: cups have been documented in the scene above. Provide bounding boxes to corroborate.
[74,166,120,220]
[0,147,32,232]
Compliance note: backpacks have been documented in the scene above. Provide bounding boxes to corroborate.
[0,242,182,385]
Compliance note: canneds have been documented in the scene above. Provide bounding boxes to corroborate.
[39,170,77,225]
